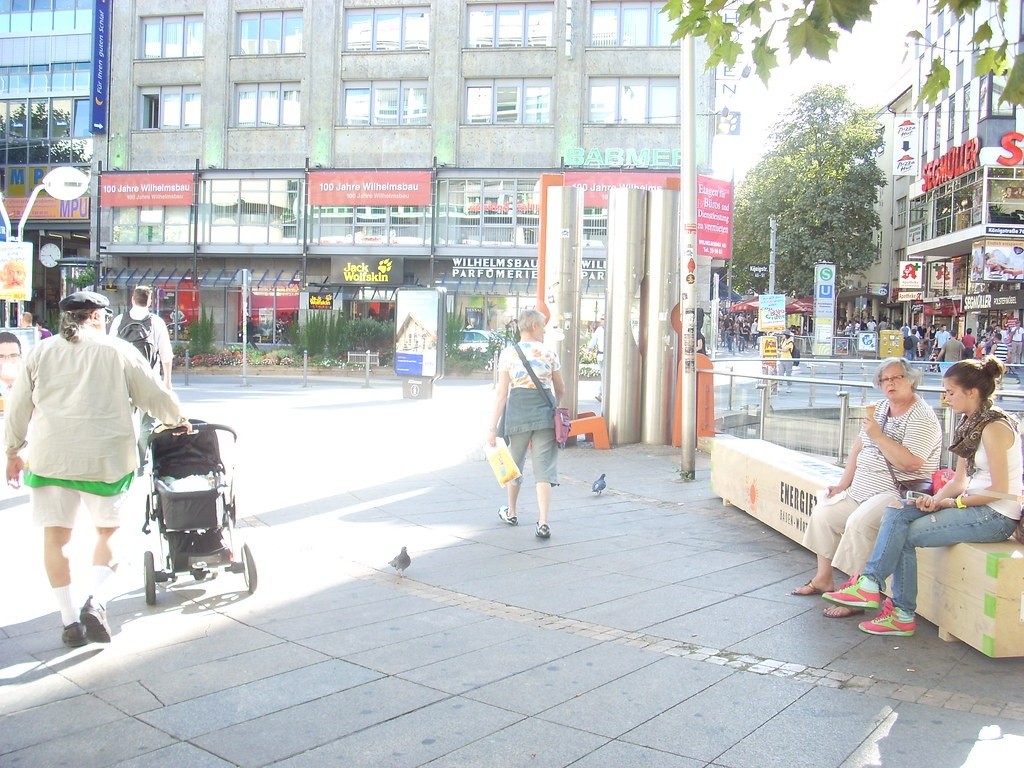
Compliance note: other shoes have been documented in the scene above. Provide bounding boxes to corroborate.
[786,386,792,393]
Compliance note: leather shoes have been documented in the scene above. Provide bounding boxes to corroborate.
[61,622,88,647]
[80,594,112,642]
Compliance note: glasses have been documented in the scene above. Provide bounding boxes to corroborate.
[0,353,22,361]
[96,314,113,324]
[879,375,904,382]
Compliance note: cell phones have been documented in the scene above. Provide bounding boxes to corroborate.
[906,491,930,501]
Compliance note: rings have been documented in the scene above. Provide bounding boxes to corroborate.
[924,503,930,509]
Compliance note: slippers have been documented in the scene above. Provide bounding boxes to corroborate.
[822,604,865,618]
[790,580,834,596]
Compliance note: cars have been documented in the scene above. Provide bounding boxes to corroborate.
[445,330,514,371]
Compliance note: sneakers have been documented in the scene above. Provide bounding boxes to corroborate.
[498,505,519,526]
[821,573,880,611]
[858,596,916,636]
[535,520,551,536]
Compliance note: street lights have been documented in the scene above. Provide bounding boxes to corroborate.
[17,167,90,327]
[834,286,852,337]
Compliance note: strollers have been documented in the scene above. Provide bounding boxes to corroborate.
[141,419,258,606]
[924,348,943,372]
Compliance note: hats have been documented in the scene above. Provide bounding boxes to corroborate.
[780,328,792,336]
[59,291,113,314]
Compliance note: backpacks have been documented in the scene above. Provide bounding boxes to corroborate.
[116,312,158,369]
[904,336,913,350]
[787,341,800,365]
[976,345,984,358]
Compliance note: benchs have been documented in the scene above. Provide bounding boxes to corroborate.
[712,435,1024,661]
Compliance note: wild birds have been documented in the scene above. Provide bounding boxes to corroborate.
[388,546,411,579]
[592,474,607,494]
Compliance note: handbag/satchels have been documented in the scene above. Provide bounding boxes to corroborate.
[555,407,570,445]
[484,438,522,488]
[895,478,934,498]
[931,469,969,494]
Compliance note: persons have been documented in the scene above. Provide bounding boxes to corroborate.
[584,313,605,401]
[988,334,1009,402]
[776,328,795,392]
[170,304,185,331]
[109,285,174,476]
[822,354,1022,636]
[237,315,258,350]
[21,312,53,344]
[511,318,521,346]
[0,332,23,397]
[720,311,1024,380]
[2,291,192,647]
[487,311,564,539]
[790,356,942,618]
[696,321,706,356]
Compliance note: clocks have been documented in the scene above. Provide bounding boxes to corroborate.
[40,244,61,267]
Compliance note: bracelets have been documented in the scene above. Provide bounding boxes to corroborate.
[949,494,967,509]
[487,424,497,431]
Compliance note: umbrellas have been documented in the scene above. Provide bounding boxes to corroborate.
[732,295,814,336]
